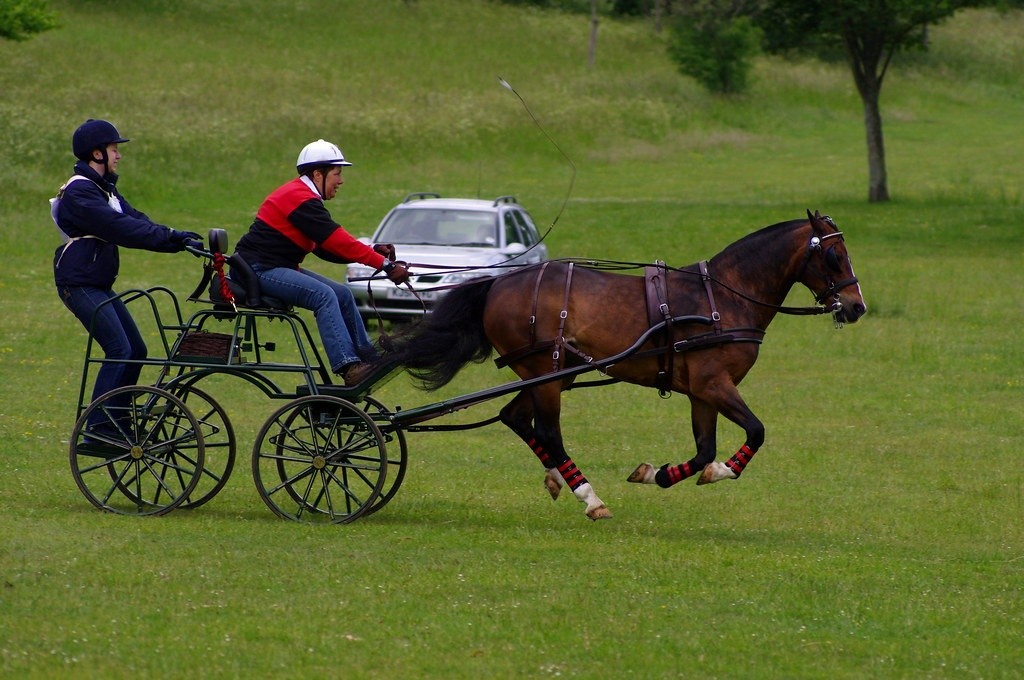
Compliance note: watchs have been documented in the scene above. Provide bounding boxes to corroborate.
[383,257,391,266]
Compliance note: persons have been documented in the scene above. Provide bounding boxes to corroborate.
[231,137,412,384]
[53,120,206,444]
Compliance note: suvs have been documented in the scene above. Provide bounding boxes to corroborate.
[344,192,547,333]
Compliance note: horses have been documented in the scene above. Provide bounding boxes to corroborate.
[361,207,869,522]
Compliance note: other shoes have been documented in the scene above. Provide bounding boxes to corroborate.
[84,418,148,449]
[373,351,392,365]
[344,361,379,387]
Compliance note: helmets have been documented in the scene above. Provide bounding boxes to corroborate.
[73,119,130,155]
[296,139,353,168]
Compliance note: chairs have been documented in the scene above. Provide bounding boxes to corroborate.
[192,228,301,320]
[478,224,493,243]
[413,220,439,241]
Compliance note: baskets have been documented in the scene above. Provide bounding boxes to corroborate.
[178,330,242,358]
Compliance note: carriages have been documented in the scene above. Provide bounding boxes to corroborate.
[68,207,870,526]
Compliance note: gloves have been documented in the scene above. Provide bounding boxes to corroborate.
[373,244,396,261]
[185,239,204,257]
[170,230,204,244]
[387,265,409,285]
